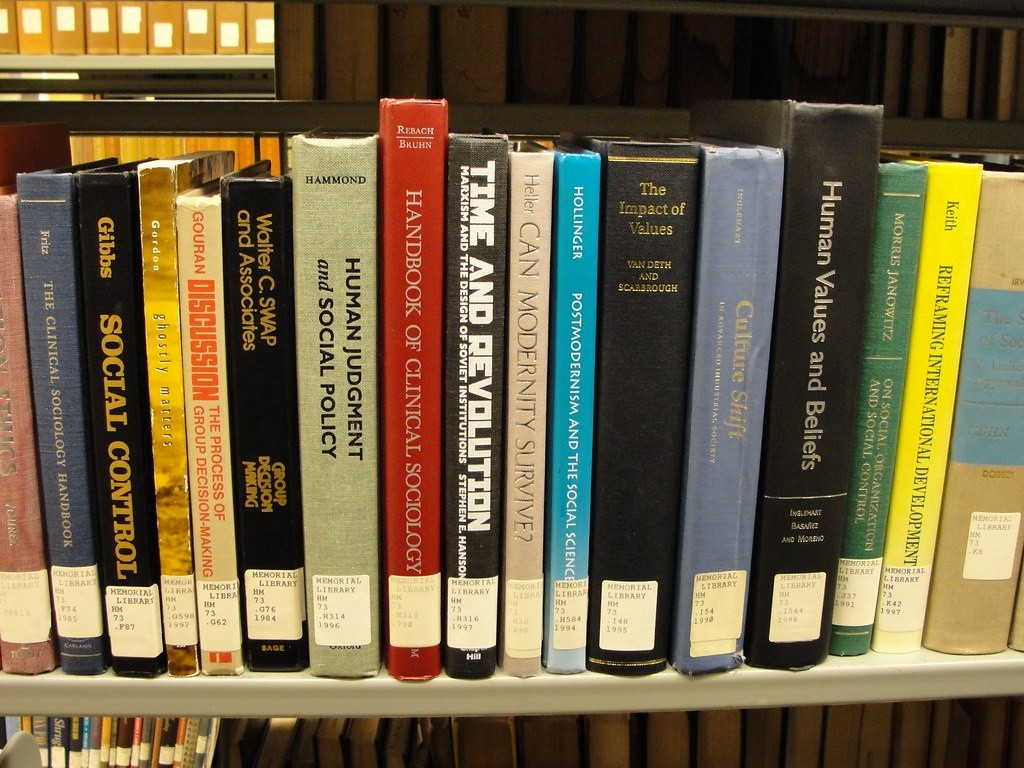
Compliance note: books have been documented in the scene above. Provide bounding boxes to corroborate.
[0,0,1024,768]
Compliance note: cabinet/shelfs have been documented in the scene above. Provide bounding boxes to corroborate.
[0,0,1024,768]
